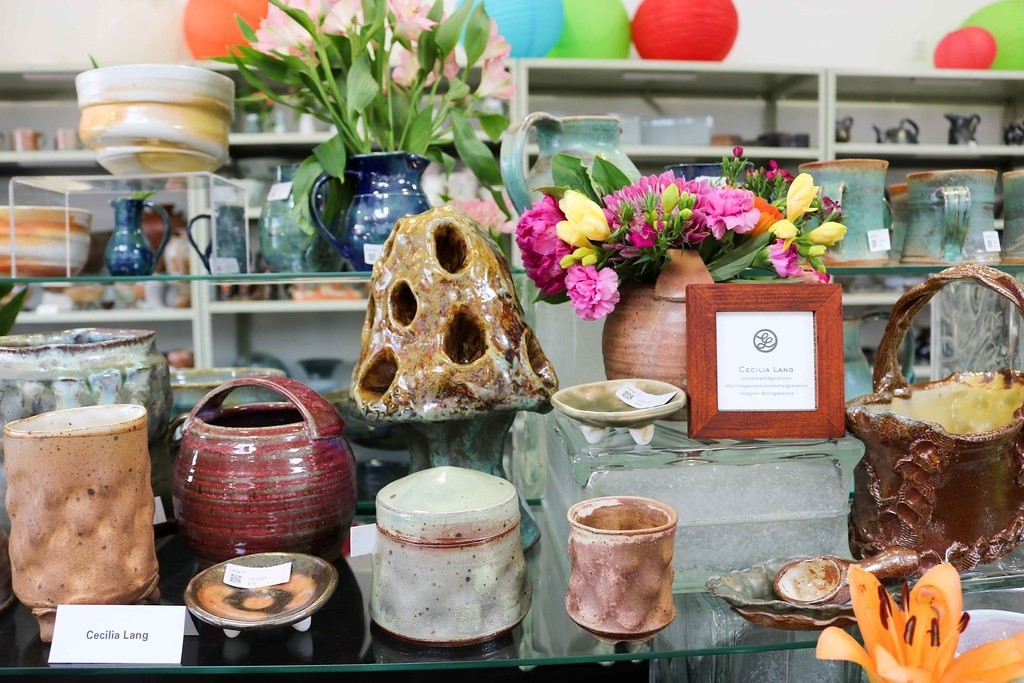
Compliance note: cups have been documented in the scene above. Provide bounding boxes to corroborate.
[299,114,315,134]
[13,129,47,151]
[55,130,79,150]
[274,111,287,132]
[566,496,678,645]
[798,158,1024,268]
[244,113,259,131]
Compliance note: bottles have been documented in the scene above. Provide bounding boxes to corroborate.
[258,165,346,273]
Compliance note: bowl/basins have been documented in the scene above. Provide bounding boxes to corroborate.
[368,467,532,648]
[75,64,235,176]
[643,117,713,146]
[0,206,93,278]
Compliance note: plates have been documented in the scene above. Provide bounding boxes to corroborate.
[185,552,339,639]
[705,555,858,632]
[551,379,687,446]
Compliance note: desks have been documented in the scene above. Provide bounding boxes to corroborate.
[0,403,1024,683]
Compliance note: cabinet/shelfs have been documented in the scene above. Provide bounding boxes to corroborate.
[0,54,1024,438]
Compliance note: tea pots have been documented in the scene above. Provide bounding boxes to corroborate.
[836,117,852,142]
[873,118,920,144]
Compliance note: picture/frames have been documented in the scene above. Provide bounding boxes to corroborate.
[686,283,846,439]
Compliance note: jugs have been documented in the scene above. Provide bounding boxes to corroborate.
[945,114,981,145]
[843,313,915,403]
[104,199,170,277]
[500,112,643,218]
[187,205,247,276]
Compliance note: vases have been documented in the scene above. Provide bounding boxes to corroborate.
[310,152,433,271]
[601,249,717,390]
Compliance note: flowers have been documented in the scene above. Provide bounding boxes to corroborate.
[204,0,515,261]
[815,562,1024,683]
[516,146,849,321]
